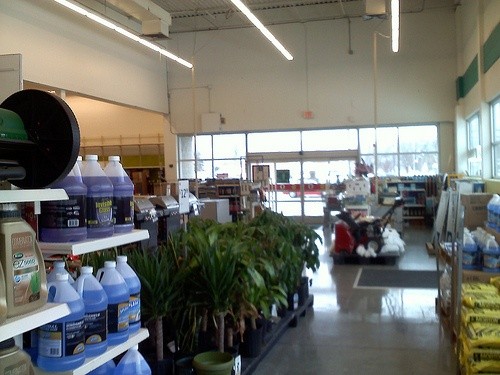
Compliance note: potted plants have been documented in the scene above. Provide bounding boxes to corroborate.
[79,208,323,375]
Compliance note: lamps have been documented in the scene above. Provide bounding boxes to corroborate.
[55,0,194,69]
[390,0,400,53]
[230,0,294,61]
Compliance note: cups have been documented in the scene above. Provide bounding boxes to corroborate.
[177,351,234,375]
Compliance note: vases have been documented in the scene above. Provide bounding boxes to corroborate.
[193,351,234,375]
[175,357,195,375]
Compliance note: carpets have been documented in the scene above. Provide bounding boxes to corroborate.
[351,268,439,291]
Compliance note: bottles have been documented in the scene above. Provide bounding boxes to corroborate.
[461,194,500,273]
[0,154,152,375]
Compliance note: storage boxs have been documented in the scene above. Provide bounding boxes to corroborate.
[459,193,500,283]
[153,180,190,214]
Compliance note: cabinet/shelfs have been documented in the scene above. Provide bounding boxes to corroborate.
[382,177,426,220]
[425,192,466,344]
[215,183,240,198]
[0,189,150,375]
